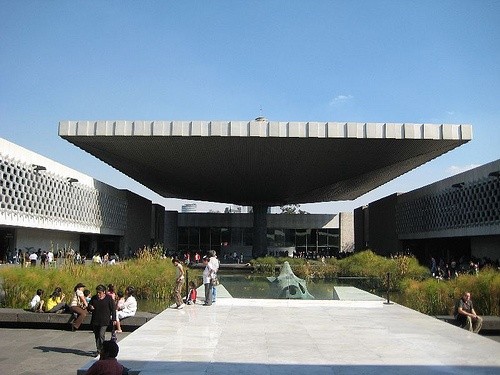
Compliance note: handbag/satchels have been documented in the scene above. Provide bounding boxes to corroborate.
[112,324,117,342]
[75,291,86,311]
[211,270,220,286]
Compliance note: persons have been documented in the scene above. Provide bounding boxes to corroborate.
[202,250,220,306]
[128,247,132,258]
[183,281,197,305]
[184,252,201,265]
[453,291,483,333]
[86,340,128,375]
[32,288,69,313]
[223,251,346,264]
[116,290,125,310]
[68,283,88,331]
[172,259,185,309]
[107,284,115,301]
[87,284,116,355]
[36,248,54,266]
[84,289,92,303]
[59,248,86,264]
[427,255,500,283]
[92,252,118,265]
[7,248,38,267]
[115,287,137,333]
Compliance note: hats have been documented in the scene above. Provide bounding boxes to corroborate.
[76,283,86,287]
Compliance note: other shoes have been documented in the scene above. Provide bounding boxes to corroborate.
[115,329,122,334]
[97,348,101,353]
[203,303,210,306]
[177,303,186,309]
[204,299,213,302]
[174,305,183,309]
[56,310,60,314]
[72,322,77,332]
[39,309,45,313]
